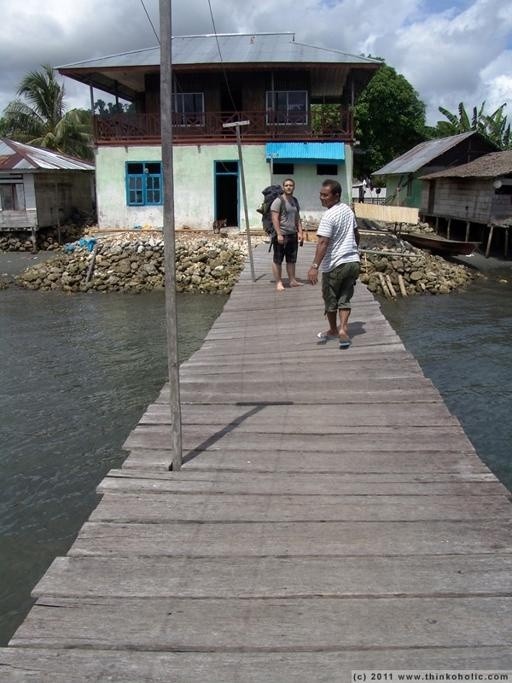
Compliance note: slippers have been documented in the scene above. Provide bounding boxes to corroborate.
[338,331,351,346]
[316,329,339,338]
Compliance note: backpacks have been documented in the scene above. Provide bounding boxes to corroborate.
[256,183,300,234]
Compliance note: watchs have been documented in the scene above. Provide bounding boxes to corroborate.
[310,262,320,270]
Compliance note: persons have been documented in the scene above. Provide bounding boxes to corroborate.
[307,178,362,347]
[270,179,305,291]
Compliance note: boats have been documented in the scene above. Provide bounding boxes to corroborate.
[396,230,483,256]
